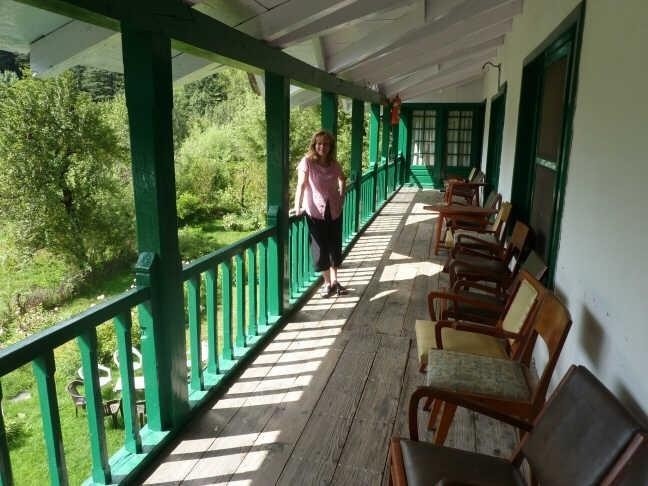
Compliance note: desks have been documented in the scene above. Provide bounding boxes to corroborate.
[115,376,146,393]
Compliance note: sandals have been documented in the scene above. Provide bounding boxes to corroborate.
[332,282,347,294]
[321,285,331,298]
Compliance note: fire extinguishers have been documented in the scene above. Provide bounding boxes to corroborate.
[391,92,401,124]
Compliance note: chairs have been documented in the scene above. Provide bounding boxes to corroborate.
[415,270,548,374]
[443,220,533,273]
[434,203,511,257]
[424,294,573,458]
[442,252,549,325]
[120,397,148,429]
[385,365,648,486]
[67,380,102,419]
[114,346,143,369]
[445,176,488,207]
[102,400,122,431]
[78,364,112,388]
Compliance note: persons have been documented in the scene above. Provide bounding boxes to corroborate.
[294,131,348,298]
